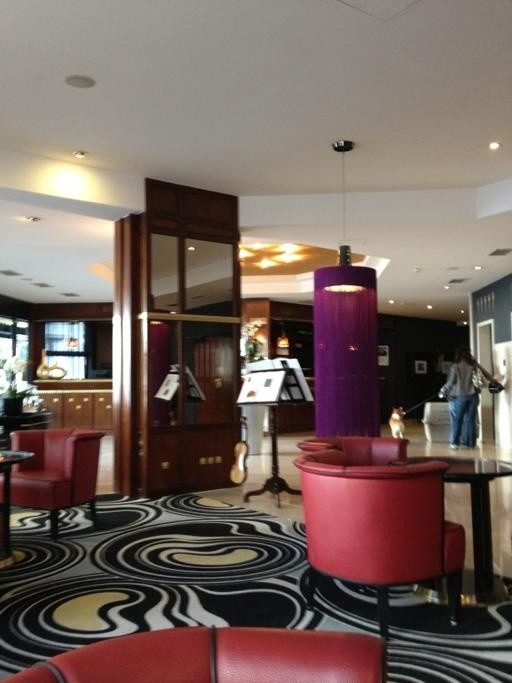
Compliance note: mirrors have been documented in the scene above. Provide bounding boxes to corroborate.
[136,217,243,433]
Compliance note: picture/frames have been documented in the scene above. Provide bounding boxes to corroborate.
[414,360,427,375]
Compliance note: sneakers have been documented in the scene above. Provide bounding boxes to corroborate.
[449,441,476,450]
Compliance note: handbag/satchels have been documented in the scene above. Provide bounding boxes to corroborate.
[472,367,489,389]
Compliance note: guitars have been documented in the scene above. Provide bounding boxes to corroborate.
[230,417,248,485]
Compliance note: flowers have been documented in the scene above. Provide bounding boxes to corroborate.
[239,319,266,342]
[0,356,39,398]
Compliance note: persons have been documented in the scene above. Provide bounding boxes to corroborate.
[438,346,500,450]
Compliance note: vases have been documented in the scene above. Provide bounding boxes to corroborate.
[4,398,22,416]
[244,337,257,363]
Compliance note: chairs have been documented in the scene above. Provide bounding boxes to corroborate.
[293,437,467,639]
[0,426,104,538]
[1,624,390,683]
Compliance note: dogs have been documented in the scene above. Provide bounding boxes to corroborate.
[388,406,407,439]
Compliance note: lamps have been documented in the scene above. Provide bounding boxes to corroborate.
[68,322,77,347]
[311,140,383,437]
[276,323,289,348]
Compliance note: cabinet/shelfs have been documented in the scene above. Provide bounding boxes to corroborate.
[37,391,113,430]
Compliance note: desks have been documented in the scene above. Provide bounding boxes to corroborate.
[392,454,512,603]
[0,450,36,551]
[0,411,56,451]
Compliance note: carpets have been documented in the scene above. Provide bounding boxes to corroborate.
[0,492,511,683]
